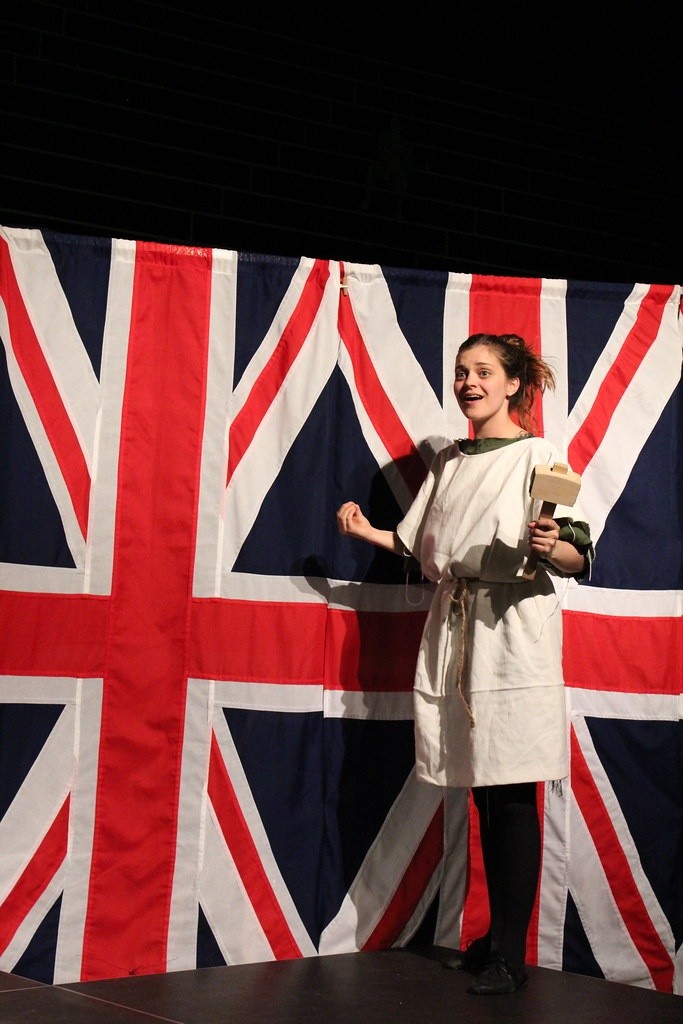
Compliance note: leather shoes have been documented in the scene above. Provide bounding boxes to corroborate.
[443,936,496,970]
[472,957,530,994]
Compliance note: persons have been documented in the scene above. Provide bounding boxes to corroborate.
[339,329,593,999]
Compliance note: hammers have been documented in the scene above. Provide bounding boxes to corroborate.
[514,461,581,583]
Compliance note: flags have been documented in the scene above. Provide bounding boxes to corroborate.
[332,235,683,1005]
[0,218,343,998]
